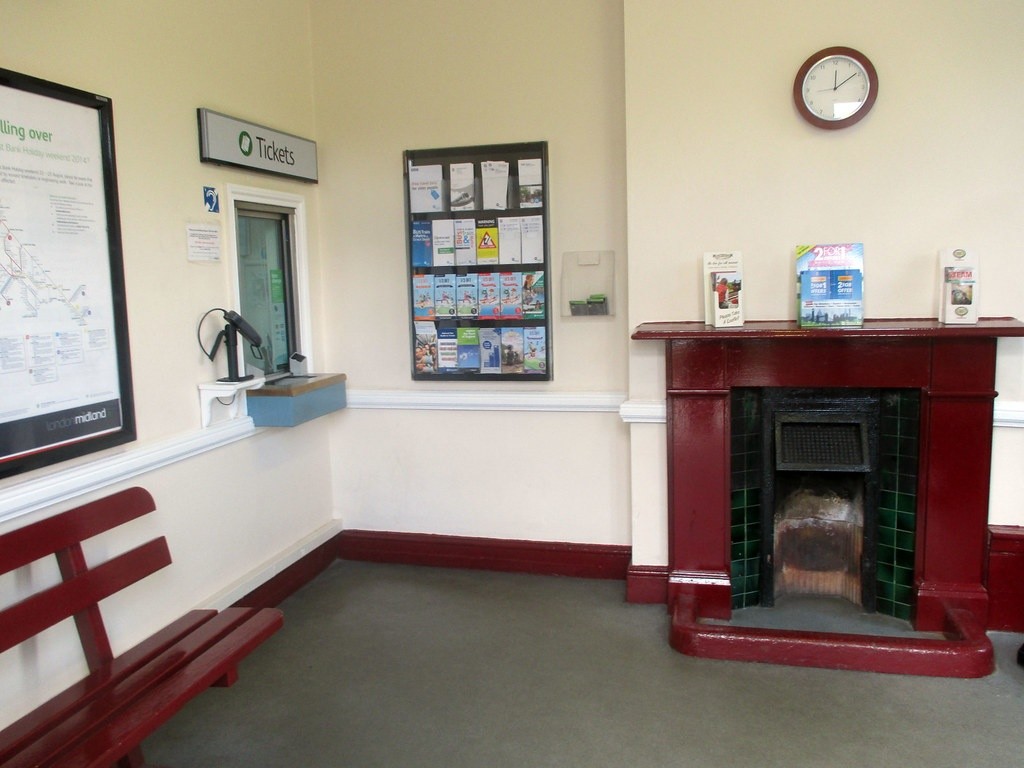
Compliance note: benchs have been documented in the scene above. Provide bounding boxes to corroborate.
[0,487,283,768]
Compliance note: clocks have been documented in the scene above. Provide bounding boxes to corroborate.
[794,46,878,130]
